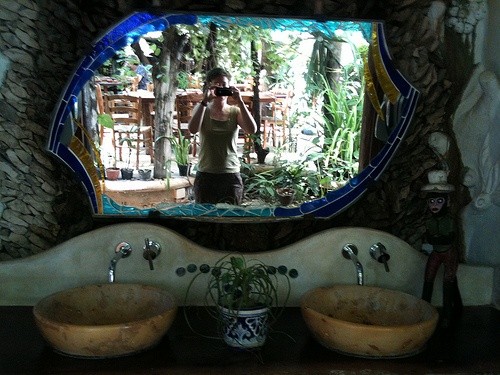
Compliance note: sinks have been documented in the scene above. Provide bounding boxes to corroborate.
[302,284,439,359]
[32,281,178,359]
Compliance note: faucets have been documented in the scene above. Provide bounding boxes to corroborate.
[105,242,131,285]
[342,242,365,287]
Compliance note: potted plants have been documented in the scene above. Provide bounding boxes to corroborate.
[177,249,291,348]
[97,113,193,180]
[276,162,304,206]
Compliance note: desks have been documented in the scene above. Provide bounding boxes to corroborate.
[98,82,120,93]
[141,97,276,155]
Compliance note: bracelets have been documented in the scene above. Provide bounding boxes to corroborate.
[200,99,208,107]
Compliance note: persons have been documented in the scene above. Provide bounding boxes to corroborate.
[187,66,257,207]
[127,59,150,91]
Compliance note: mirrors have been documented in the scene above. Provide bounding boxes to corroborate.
[44,11,421,224]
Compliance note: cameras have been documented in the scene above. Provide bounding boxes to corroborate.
[214,87,233,97]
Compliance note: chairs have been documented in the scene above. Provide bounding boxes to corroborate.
[92,83,291,169]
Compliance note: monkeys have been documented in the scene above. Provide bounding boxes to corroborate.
[421,190,469,321]
